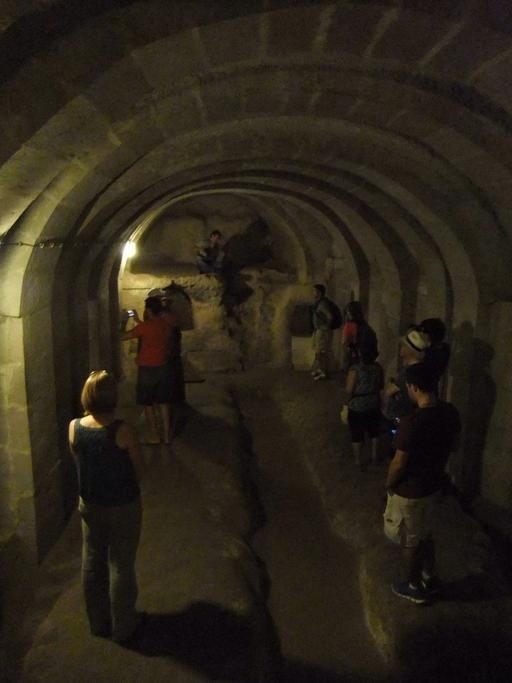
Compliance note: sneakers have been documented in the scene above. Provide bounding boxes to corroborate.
[390,574,440,605]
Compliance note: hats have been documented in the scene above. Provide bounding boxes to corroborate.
[400,330,430,360]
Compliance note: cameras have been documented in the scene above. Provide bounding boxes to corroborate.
[385,416,400,438]
[126,310,136,317]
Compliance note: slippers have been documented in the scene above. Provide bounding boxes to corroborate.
[138,432,174,445]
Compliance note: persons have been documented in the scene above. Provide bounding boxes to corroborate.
[115,285,186,444]
[195,229,225,274]
[68,368,144,643]
[340,300,384,467]
[311,284,334,380]
[383,318,459,604]
